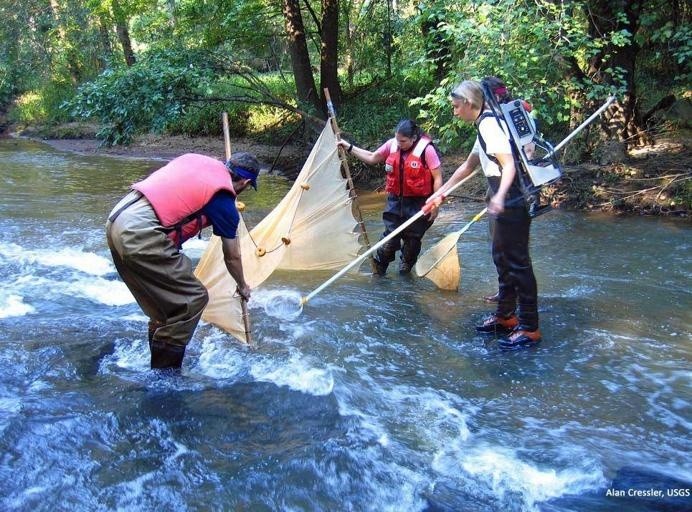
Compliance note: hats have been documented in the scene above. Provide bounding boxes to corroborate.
[226,161,258,193]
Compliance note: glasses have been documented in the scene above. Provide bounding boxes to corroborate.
[451,90,464,99]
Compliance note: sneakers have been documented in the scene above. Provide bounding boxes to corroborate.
[484,289,521,303]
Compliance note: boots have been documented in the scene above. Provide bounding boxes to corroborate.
[149,330,186,369]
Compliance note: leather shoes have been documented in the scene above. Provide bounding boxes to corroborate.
[497,327,542,352]
[474,314,519,336]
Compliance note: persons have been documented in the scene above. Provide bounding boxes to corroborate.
[106,151,260,371]
[340,117,442,275]
[426,79,542,348]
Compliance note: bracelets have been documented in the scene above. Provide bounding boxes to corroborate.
[347,144,353,152]
